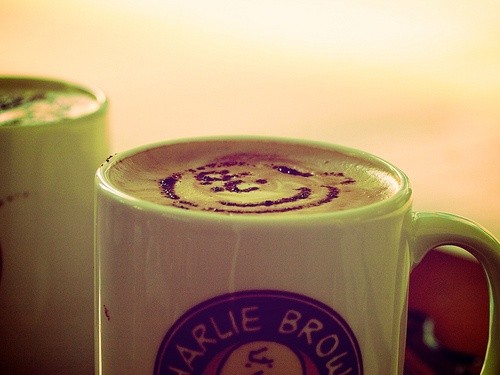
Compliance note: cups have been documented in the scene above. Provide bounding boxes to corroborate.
[94,135,499,375]
[0,75,110,374]
